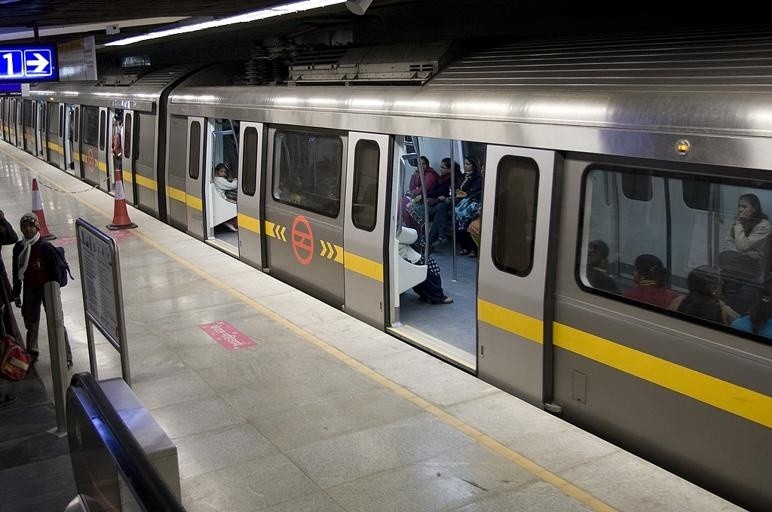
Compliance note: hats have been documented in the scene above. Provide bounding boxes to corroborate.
[688,264,721,288]
[19,212,41,230]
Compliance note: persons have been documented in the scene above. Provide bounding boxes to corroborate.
[620,254,682,308]
[731,281,771,339]
[415,157,462,232]
[213,163,238,232]
[669,266,740,326]
[114,121,123,170]
[457,161,484,258]
[422,153,482,255]
[720,193,772,316]
[0,211,17,407]
[13,213,72,369]
[496,175,529,262]
[589,240,620,294]
[396,225,453,304]
[399,156,439,228]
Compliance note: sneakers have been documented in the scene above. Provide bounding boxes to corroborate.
[431,236,477,258]
[224,222,239,232]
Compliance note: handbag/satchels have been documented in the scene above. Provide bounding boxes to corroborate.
[0,334,35,381]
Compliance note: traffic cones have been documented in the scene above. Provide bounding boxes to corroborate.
[28,177,56,243]
[105,168,137,231]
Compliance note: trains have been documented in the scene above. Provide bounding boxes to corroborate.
[1,12,771,512]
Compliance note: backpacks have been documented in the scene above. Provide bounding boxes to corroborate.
[36,242,75,288]
